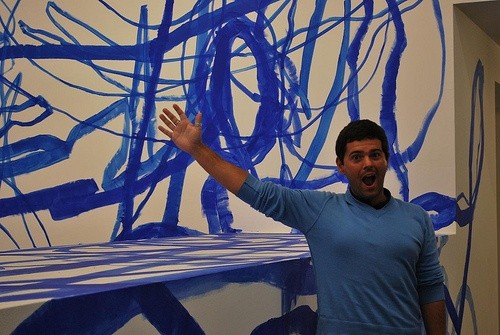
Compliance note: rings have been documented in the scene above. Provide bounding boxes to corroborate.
[175,120,180,125]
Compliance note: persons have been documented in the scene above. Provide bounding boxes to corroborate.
[158,104,447,335]
